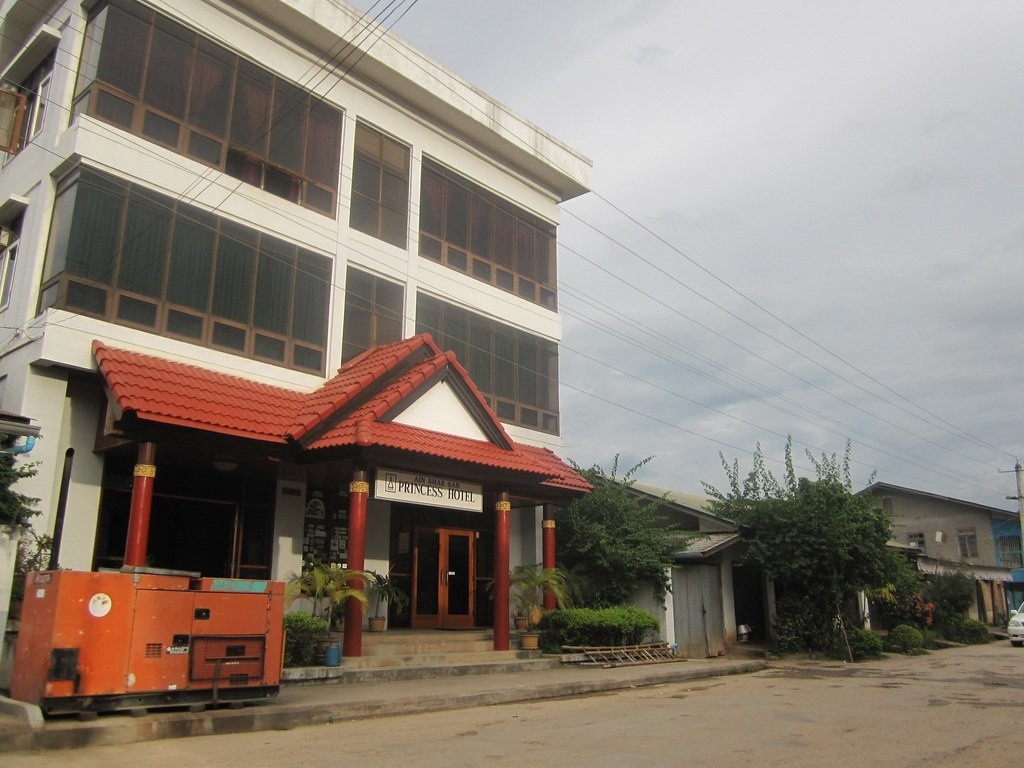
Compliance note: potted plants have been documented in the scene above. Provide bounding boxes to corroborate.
[329,569,351,631]
[515,585,539,649]
[510,592,528,630]
[284,564,373,657]
[485,563,569,626]
[366,570,409,631]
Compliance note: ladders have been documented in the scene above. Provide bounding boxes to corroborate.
[560,641,674,667]
[584,646,688,669]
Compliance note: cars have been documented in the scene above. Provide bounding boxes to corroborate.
[1007,601,1024,647]
